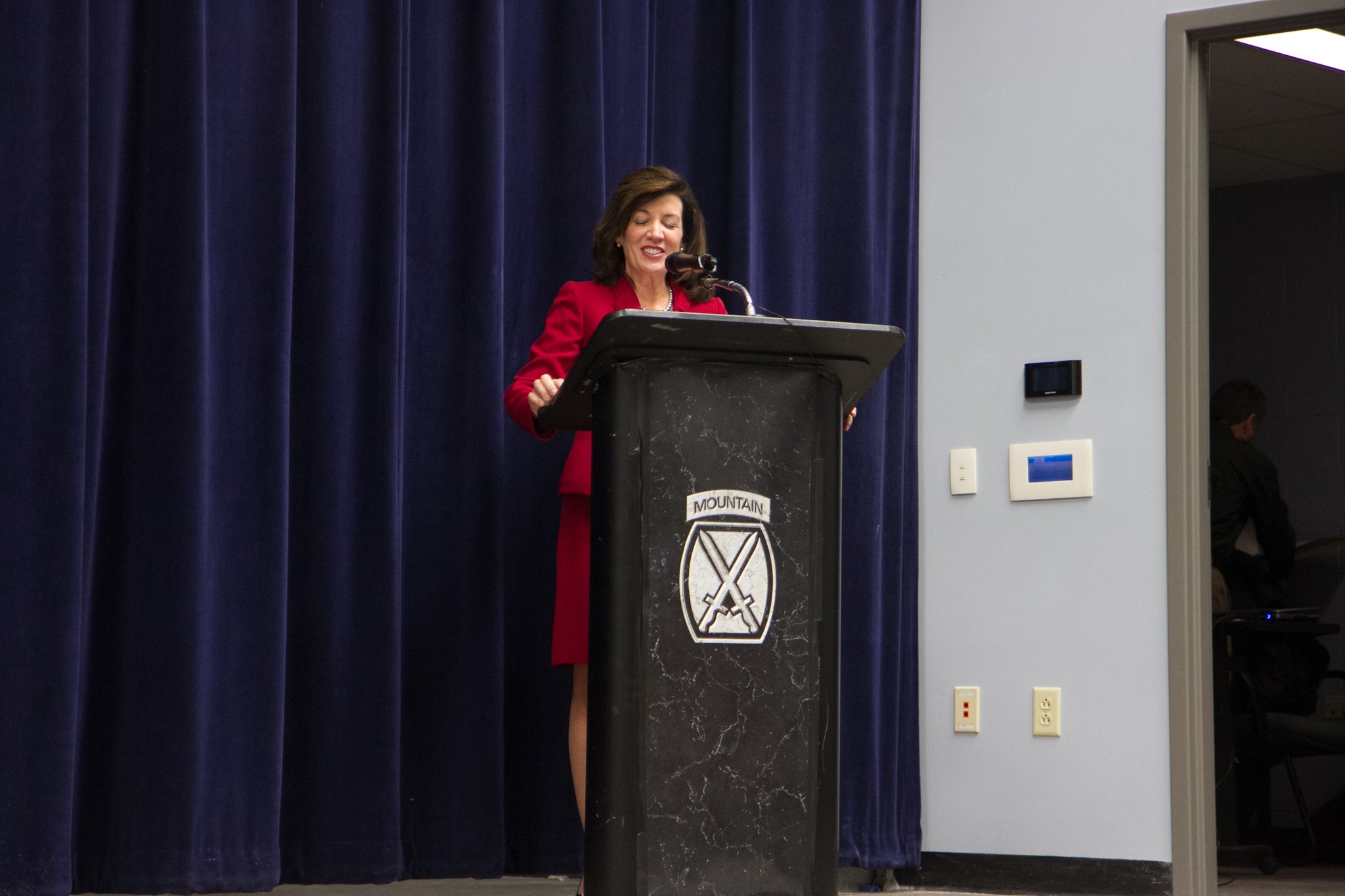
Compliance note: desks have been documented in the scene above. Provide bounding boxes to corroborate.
[1217,616,1341,875]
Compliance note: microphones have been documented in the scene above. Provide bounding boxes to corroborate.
[665,251,717,276]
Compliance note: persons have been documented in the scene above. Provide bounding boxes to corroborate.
[503,165,857,896]
[1209,377,1300,851]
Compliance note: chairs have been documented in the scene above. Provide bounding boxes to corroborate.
[1214,534,1345,854]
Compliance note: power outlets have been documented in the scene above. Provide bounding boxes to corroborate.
[1032,687,1061,738]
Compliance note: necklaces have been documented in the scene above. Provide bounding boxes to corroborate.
[641,279,675,311]
